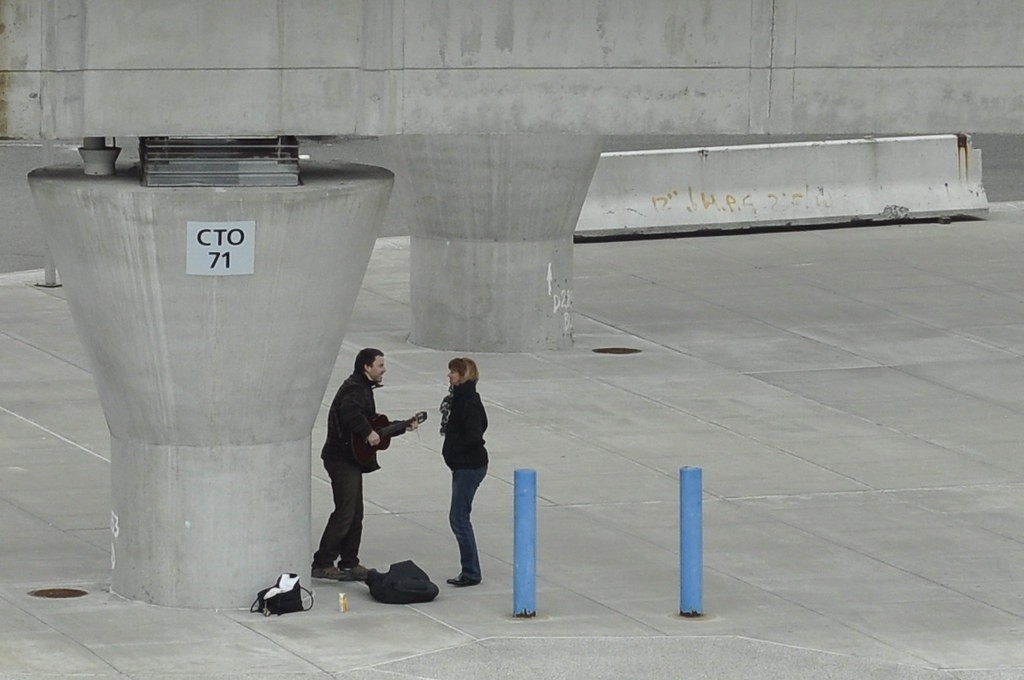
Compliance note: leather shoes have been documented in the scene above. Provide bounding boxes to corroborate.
[446,571,480,586]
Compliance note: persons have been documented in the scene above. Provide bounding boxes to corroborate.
[312,348,418,580]
[440,358,490,587]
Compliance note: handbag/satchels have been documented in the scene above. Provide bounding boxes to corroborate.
[249,572,313,617]
[365,560,439,604]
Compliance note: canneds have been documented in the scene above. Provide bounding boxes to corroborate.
[338,592,348,612]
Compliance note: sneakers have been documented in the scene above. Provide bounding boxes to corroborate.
[311,564,348,581]
[337,561,371,582]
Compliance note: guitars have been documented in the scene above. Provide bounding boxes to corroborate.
[345,410,428,465]
